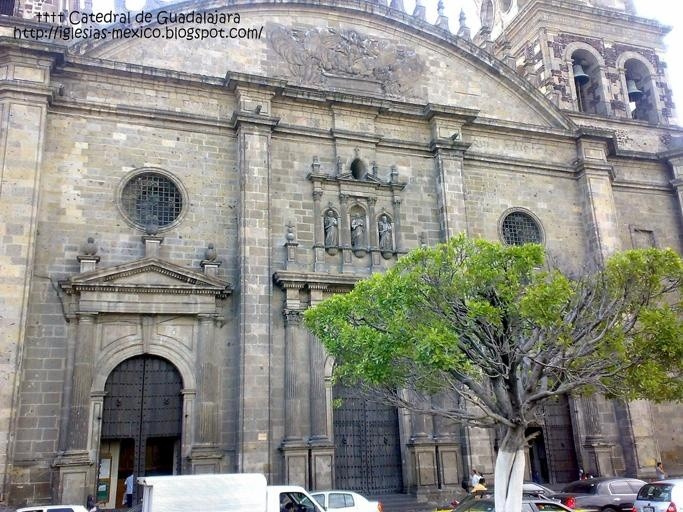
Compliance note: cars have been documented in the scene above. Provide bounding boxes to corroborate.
[299,486,384,512]
[630,479,682,512]
[125,504,141,512]
[428,477,579,512]
[15,504,89,512]
[544,476,649,512]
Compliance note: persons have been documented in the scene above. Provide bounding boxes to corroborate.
[87,495,97,512]
[533,470,541,485]
[284,502,294,512]
[477,471,485,479]
[471,478,487,493]
[470,469,480,488]
[655,462,666,480]
[123,472,133,508]
[324,210,338,247]
[352,212,366,247]
[379,216,392,250]
[576,466,596,481]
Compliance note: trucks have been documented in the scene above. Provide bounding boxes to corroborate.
[136,472,326,512]
